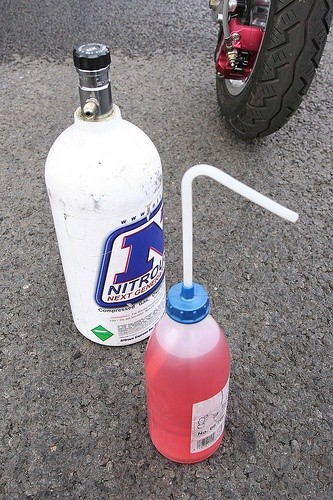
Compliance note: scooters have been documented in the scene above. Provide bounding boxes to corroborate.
[208,0,333,144]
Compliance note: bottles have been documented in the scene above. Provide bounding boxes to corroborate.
[143,164,300,466]
[44,42,167,347]
